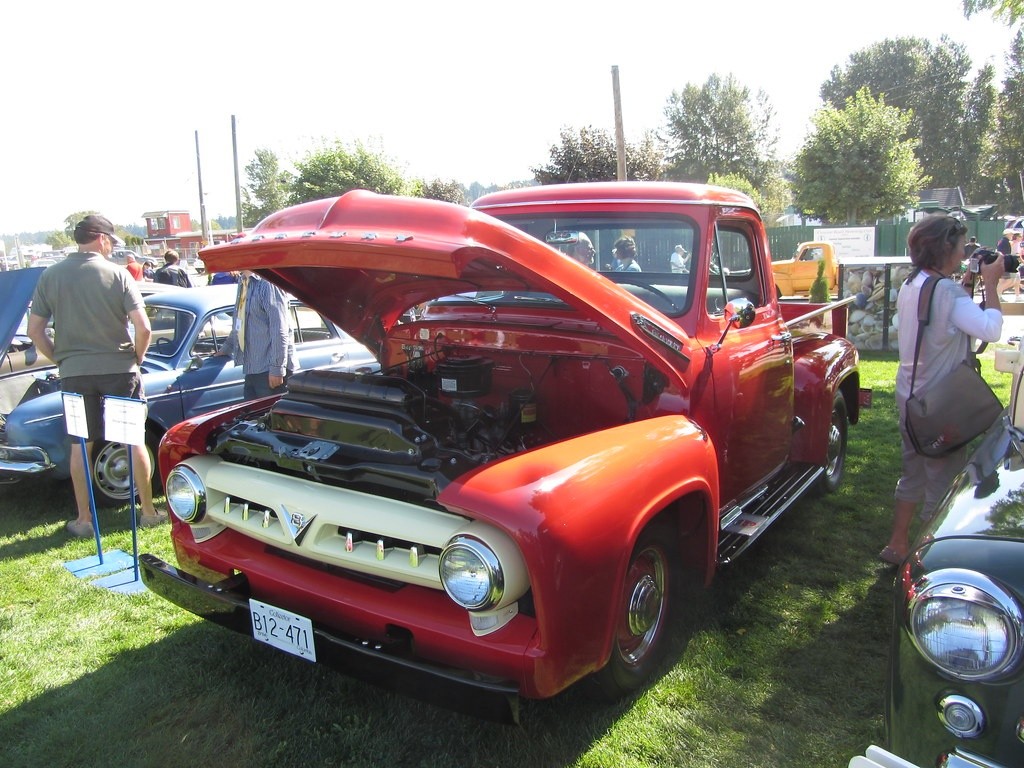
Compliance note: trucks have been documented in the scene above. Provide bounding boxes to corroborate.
[770,241,913,301]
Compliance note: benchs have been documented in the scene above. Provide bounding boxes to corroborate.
[615,283,759,316]
[294,330,331,343]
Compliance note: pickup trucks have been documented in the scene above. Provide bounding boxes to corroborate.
[134,177,874,726]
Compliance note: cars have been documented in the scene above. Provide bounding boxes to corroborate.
[0,248,382,509]
[848,332,1024,768]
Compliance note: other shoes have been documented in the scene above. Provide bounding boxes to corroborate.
[880,546,901,566]
[1015,297,1024,302]
[67,519,95,540]
[1000,297,1009,302]
[141,509,168,527]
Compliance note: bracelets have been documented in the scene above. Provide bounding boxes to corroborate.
[962,282,972,287]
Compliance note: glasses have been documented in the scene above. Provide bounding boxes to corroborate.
[947,215,962,239]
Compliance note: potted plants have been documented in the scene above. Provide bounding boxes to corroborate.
[808,261,830,326]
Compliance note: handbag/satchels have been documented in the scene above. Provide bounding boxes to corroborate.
[906,354,1005,458]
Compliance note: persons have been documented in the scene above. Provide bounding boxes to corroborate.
[670,245,691,274]
[997,229,1015,302]
[212,270,302,402]
[211,270,241,285]
[1004,232,1024,301]
[964,236,980,261]
[606,235,643,272]
[142,260,155,281]
[28,214,170,537]
[126,254,144,281]
[566,231,596,265]
[153,250,192,288]
[879,216,1004,565]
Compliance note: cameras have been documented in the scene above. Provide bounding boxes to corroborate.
[973,249,1020,273]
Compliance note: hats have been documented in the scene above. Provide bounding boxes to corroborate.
[1003,229,1018,234]
[75,214,126,246]
